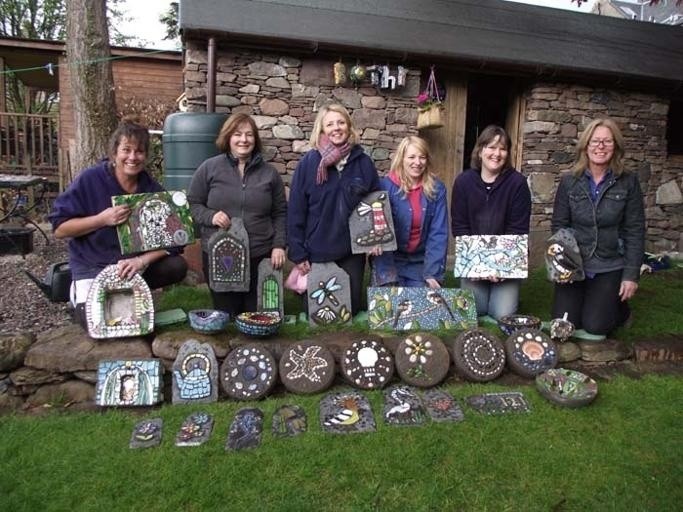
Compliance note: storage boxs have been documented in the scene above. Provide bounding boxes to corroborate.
[0,225,37,254]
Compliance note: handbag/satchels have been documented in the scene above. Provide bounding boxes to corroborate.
[648,253,671,270]
[640,252,656,266]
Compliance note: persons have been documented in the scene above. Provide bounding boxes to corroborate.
[287,103,378,320]
[366,136,449,289]
[550,118,645,335]
[449,125,532,322]
[48,123,188,328]
[186,112,289,320]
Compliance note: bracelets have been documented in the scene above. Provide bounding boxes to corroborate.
[136,255,146,268]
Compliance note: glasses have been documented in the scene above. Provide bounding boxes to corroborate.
[589,139,615,146]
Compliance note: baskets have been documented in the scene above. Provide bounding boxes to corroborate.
[414,70,445,131]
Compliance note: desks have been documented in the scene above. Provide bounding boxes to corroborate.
[0,173,53,259]
